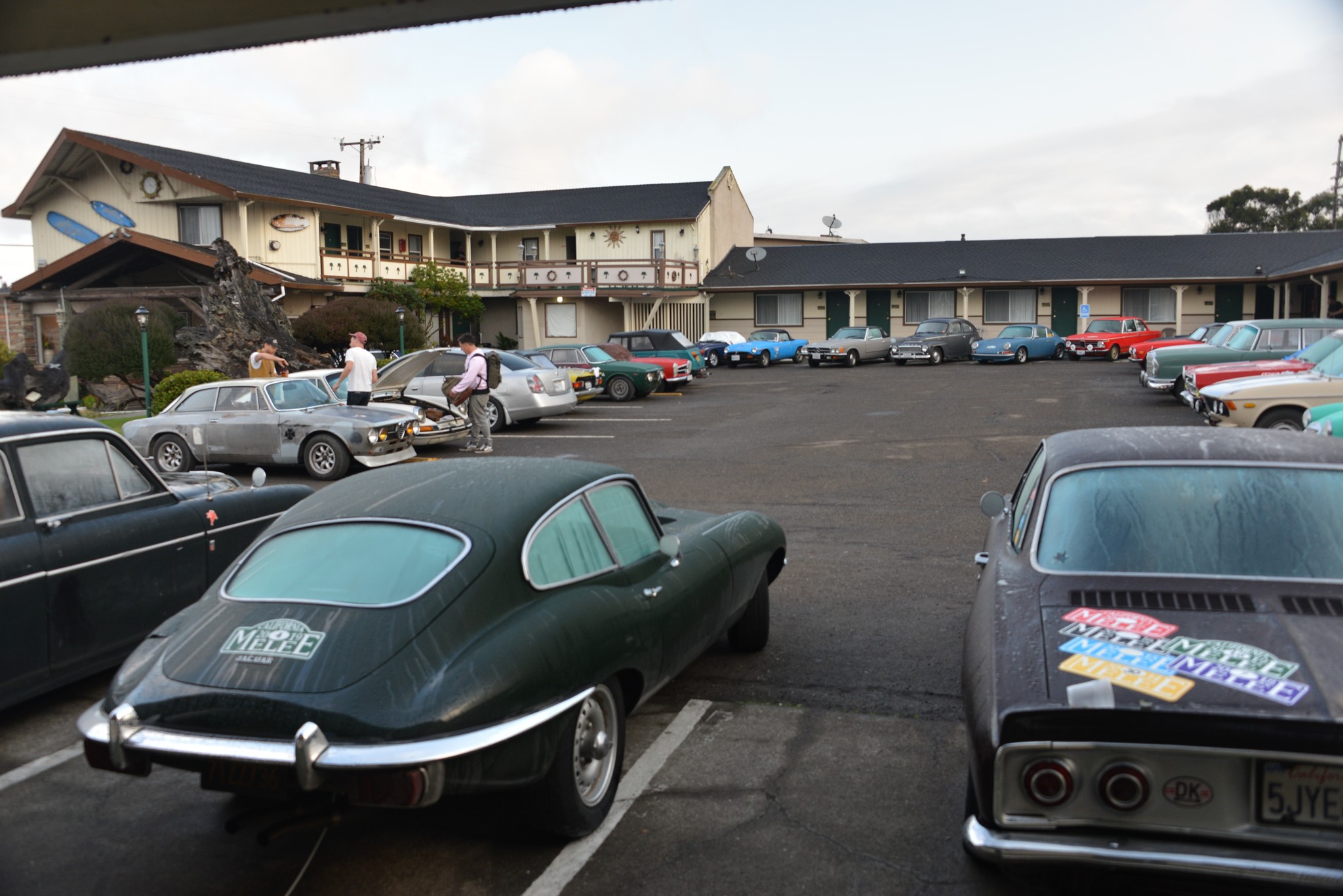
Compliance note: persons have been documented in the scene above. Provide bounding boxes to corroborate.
[248,337,289,407]
[333,332,378,406]
[450,333,493,453]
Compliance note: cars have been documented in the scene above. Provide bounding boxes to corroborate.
[231,369,474,446]
[800,326,906,368]
[694,331,747,368]
[722,328,810,369]
[1,407,315,717]
[971,324,1066,365]
[373,347,579,436]
[502,342,693,405]
[122,377,427,483]
[889,318,985,367]
[1127,318,1343,442]
[960,425,1343,896]
[1065,317,1164,362]
[606,328,705,377]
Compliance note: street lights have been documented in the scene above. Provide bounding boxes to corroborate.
[134,305,151,418]
[395,306,406,356]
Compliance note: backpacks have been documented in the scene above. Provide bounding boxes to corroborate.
[466,351,502,389]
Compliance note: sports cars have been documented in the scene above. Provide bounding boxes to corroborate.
[75,451,790,843]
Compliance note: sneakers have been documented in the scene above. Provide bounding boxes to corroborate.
[474,443,493,454]
[459,441,479,451]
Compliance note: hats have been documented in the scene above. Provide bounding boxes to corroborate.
[348,332,367,344]
[263,336,282,348]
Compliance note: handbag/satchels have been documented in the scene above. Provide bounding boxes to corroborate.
[440,376,472,408]
